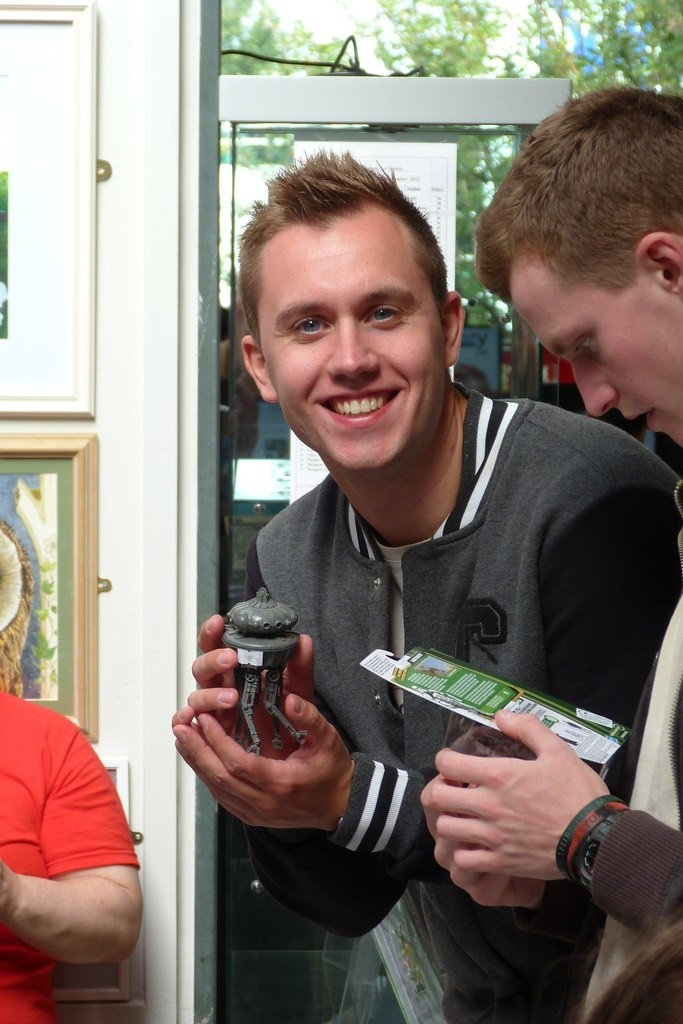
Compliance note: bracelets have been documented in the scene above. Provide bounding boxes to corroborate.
[556,795,630,885]
[339,816,343,824]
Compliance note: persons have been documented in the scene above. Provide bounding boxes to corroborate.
[171,146,683,1024]
[0,690,143,1024]
[420,89,683,1024]
[581,914,683,1024]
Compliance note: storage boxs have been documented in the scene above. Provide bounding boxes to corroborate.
[357,649,631,796]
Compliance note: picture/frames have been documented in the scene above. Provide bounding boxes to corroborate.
[34,760,133,1000]
[0,0,100,417]
[1,409,97,736]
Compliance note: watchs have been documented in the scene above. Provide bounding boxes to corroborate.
[573,809,633,892]
